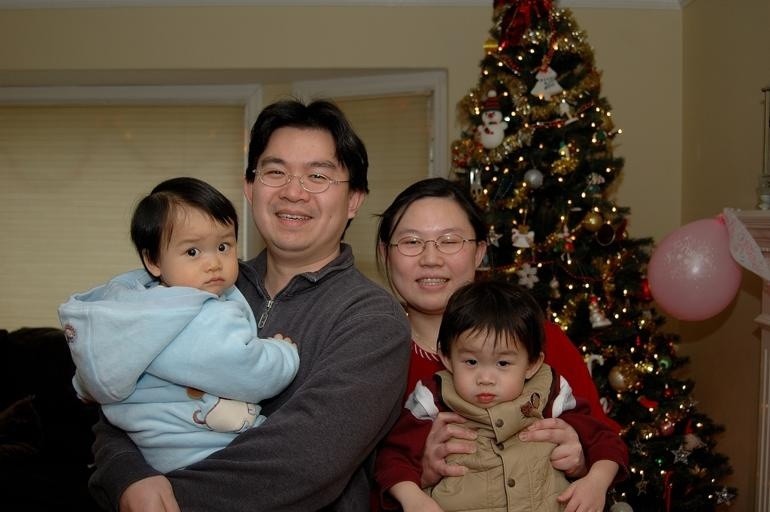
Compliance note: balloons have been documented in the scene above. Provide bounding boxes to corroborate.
[647,213,743,322]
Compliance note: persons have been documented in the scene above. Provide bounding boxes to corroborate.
[373,283,628,512]
[372,176,631,510]
[58,179,300,474]
[87,94,411,511]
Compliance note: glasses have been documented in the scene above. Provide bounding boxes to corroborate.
[252,168,350,193]
[387,233,478,257]
